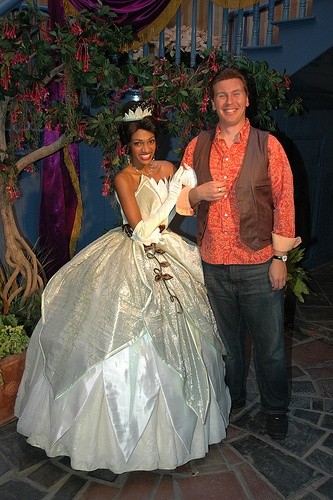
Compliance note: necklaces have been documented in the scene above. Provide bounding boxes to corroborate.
[128,160,154,176]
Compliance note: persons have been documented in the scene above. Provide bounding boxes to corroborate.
[175,67,303,440]
[13,102,230,475]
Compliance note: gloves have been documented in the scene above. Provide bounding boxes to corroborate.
[180,162,197,188]
[131,165,186,242]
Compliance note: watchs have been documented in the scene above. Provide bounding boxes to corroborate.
[273,254,288,262]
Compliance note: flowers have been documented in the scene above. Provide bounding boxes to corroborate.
[149,25,219,59]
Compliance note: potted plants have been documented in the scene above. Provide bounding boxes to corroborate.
[0,0,310,430]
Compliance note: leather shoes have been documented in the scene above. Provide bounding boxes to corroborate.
[266,414,287,439]
[231,402,246,409]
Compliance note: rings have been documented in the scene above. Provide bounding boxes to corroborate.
[218,188,222,192]
